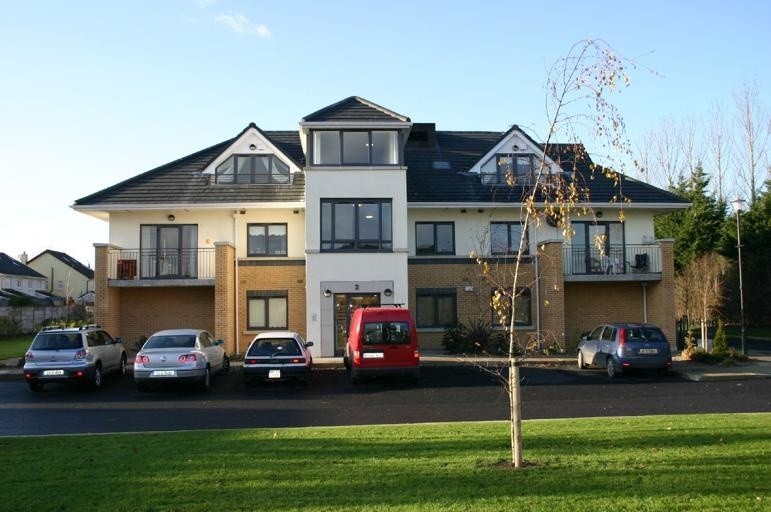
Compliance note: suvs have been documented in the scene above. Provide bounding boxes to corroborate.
[22,322,129,393]
[574,320,674,379]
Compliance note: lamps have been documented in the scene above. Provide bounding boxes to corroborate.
[168,214,175,221]
[384,288,393,296]
[324,289,332,298]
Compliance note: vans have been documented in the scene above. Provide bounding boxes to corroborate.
[342,303,420,382]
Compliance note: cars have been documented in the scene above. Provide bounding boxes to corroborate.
[242,330,314,389]
[132,328,231,390]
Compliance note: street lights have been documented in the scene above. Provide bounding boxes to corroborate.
[729,195,749,358]
